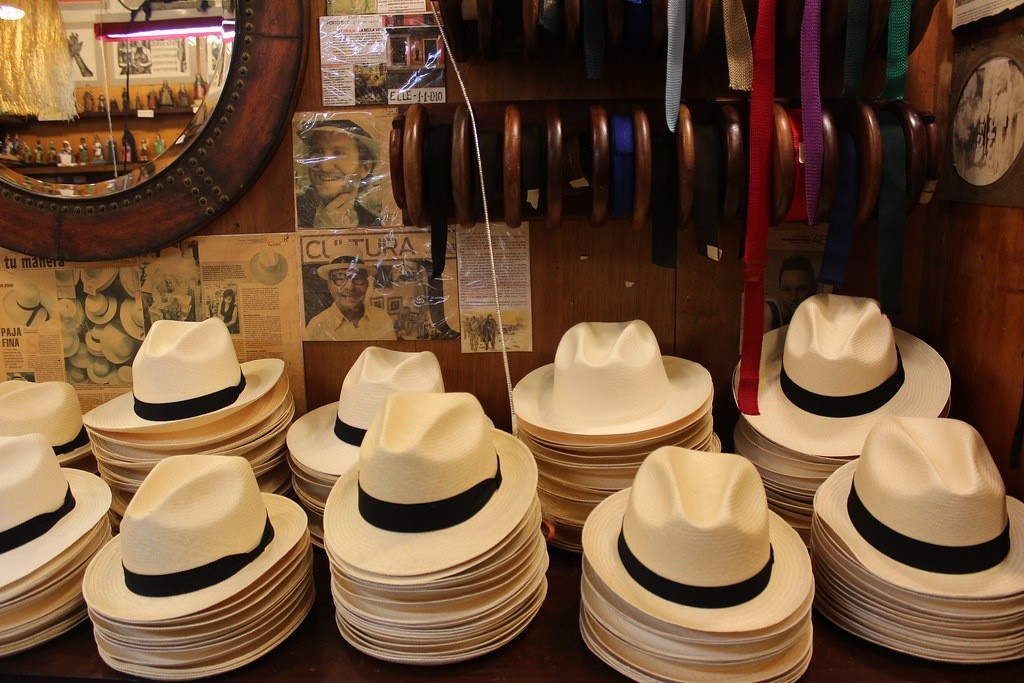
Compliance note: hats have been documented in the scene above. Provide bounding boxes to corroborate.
[0,436,113,655]
[730,293,952,548]
[4,282,53,328]
[322,392,548,664]
[510,321,722,553]
[297,113,381,165]
[316,247,378,280]
[284,346,446,546]
[0,379,98,474]
[82,455,316,679]
[579,444,812,683]
[84,318,296,534]
[810,417,1024,663]
[251,250,288,286]
[56,265,146,386]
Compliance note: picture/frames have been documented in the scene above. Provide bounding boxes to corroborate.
[947,27,1024,207]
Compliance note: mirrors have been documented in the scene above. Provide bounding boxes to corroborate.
[0,0,308,261]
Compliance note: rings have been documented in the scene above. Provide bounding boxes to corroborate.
[346,212,356,223]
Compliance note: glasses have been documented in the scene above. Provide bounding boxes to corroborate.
[330,272,367,287]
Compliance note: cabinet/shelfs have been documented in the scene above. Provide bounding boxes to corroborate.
[0,103,204,176]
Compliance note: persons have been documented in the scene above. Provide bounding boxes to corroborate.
[762,257,815,338]
[216,288,237,335]
[296,119,382,227]
[303,255,395,341]
[0,71,207,171]
[463,314,500,351]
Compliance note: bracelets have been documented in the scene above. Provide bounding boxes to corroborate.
[397,0,929,234]
